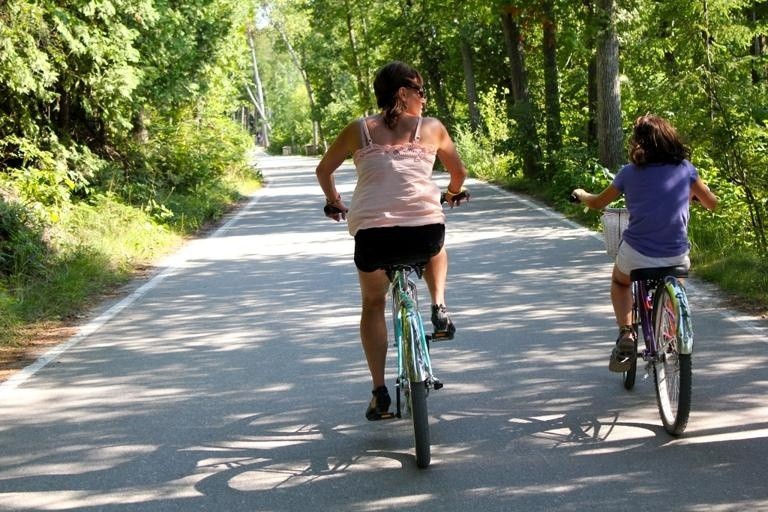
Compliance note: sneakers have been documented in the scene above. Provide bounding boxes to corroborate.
[431,304,455,335]
[366,385,391,420]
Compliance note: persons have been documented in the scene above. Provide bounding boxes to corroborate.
[577,114,718,372]
[316,61,468,419]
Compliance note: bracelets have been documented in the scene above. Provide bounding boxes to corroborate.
[327,195,341,206]
[448,189,463,196]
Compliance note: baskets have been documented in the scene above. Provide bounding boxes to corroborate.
[600,212,630,257]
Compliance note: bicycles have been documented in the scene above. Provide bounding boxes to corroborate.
[322,191,472,469]
[571,191,707,437]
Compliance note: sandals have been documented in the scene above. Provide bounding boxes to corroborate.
[609,325,635,372]
